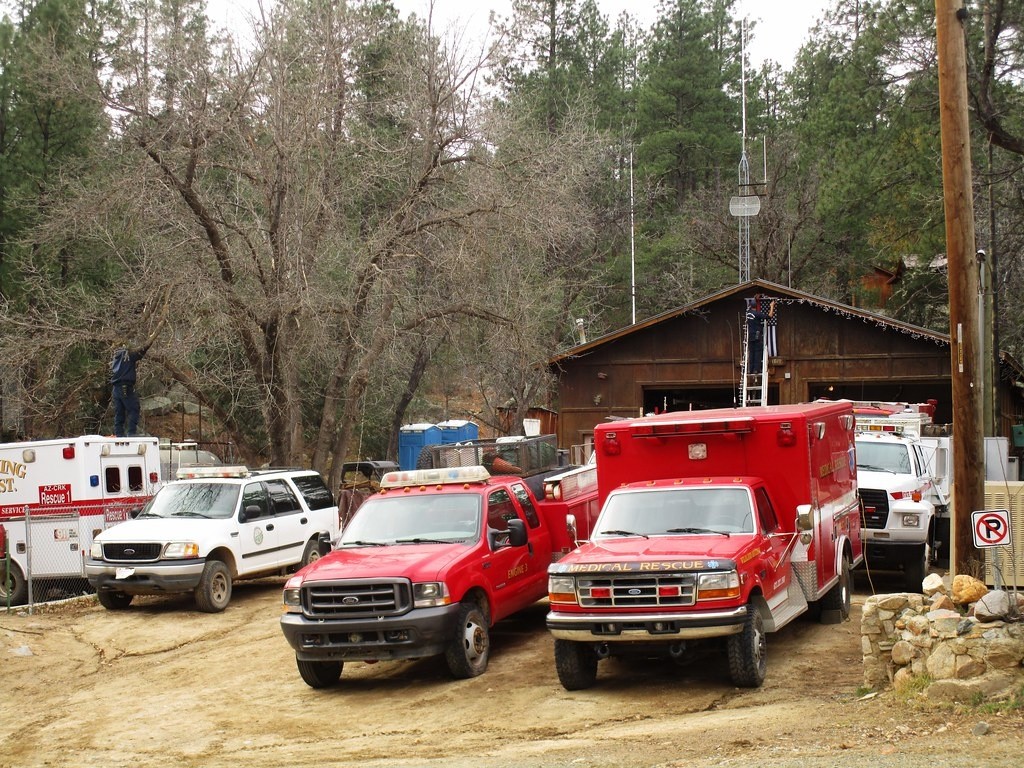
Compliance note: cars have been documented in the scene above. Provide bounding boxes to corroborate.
[158,449,225,481]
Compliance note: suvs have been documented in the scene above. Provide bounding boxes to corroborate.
[85,465,340,613]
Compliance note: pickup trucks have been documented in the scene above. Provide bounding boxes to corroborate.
[280,432,599,691]
[545,402,862,692]
[811,395,1009,592]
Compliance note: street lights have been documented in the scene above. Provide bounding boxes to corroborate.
[974,249,988,488]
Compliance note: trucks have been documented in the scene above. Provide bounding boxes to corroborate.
[0,435,162,608]
